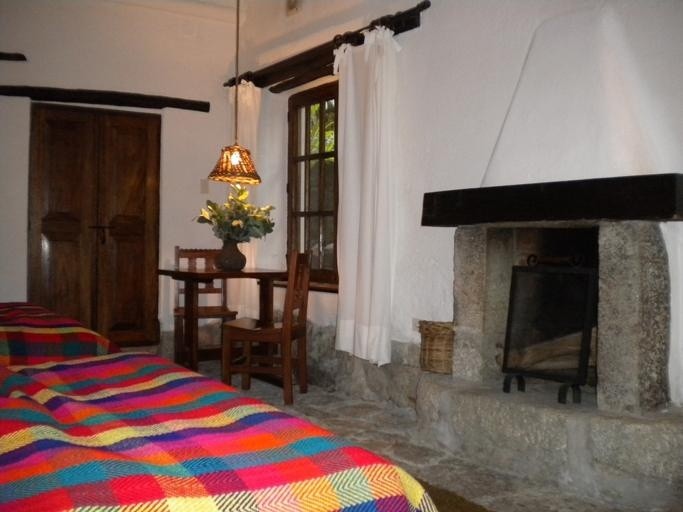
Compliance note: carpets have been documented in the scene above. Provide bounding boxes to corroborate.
[412,476,491,511]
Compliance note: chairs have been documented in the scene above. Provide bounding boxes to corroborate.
[171,245,314,405]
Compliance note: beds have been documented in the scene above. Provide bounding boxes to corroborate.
[1,299,437,512]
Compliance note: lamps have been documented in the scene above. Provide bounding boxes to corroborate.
[208,0,262,189]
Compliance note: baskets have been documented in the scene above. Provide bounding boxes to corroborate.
[418,319,455,376]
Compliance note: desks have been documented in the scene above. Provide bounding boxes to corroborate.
[155,264,290,390]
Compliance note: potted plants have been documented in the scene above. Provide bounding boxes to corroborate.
[190,182,278,272]
